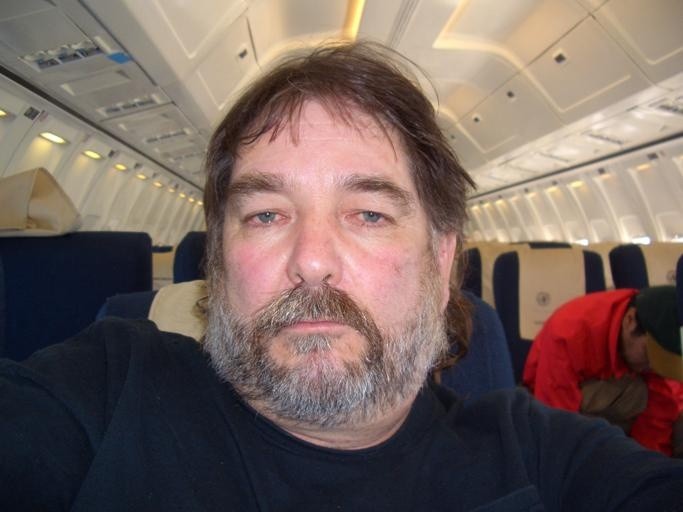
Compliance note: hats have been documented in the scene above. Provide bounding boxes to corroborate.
[638,286,683,379]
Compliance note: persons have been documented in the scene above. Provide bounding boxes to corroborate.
[523,285,683,456]
[0,25,682,512]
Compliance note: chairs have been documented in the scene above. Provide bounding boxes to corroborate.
[0,232,683,512]
